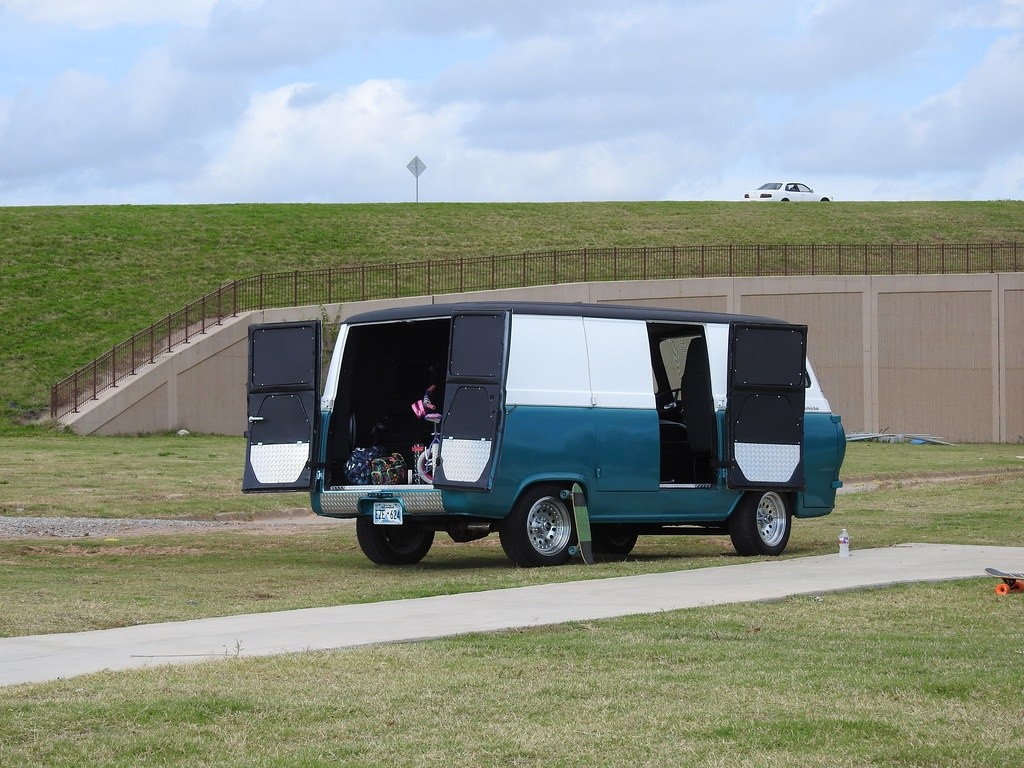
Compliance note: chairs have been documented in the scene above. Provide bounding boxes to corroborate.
[659,419,689,475]
[681,337,715,483]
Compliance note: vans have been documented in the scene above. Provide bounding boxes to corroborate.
[238,300,846,567]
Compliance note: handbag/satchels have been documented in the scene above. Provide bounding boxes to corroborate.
[343,445,389,484]
[371,452,407,485]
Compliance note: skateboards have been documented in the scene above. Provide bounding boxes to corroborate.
[983,568,1024,596]
[560,483,594,565]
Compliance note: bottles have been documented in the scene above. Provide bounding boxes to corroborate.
[839,529,849,557]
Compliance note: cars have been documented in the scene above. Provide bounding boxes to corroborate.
[744,182,832,202]
